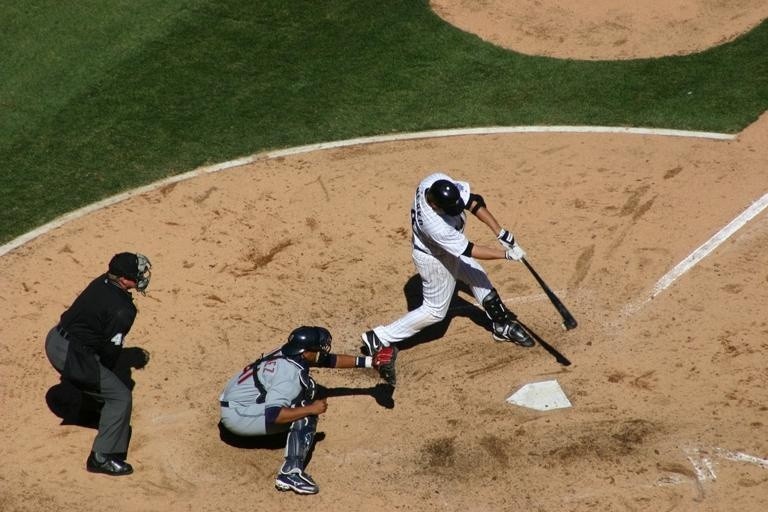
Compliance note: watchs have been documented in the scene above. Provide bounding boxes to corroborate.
[218,325,402,496]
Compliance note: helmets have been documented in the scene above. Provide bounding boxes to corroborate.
[279,324,322,358]
[425,179,466,217]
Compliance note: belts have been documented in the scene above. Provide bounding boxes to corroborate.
[55,321,73,342]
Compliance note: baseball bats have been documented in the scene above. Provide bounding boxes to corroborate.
[520,257,578,330]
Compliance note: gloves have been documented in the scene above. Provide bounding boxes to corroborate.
[495,227,519,250]
[505,246,527,264]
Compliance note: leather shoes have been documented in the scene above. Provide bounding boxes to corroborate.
[86,450,134,478]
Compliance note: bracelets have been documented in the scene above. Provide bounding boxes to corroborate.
[356,355,374,368]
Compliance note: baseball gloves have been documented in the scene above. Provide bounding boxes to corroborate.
[371,346,398,384]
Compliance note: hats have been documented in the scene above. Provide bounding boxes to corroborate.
[107,251,141,283]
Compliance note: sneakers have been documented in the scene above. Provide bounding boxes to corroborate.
[274,467,321,496]
[491,321,536,349]
[360,328,386,357]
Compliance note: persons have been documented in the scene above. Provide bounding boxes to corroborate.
[361,172,535,356]
[44,250,154,476]
